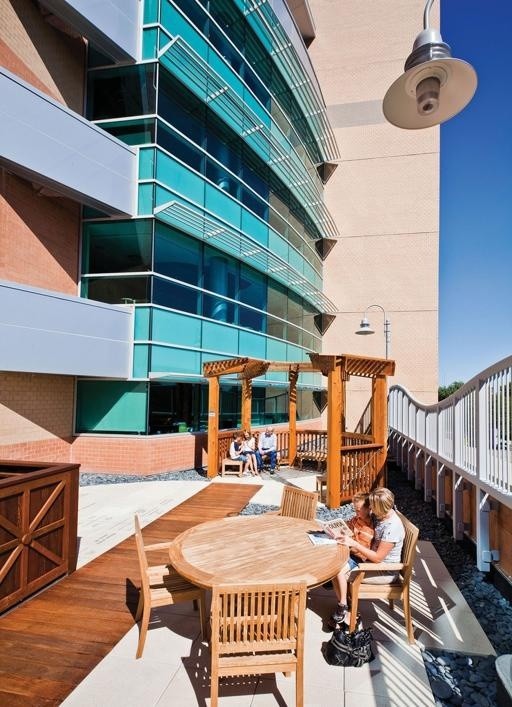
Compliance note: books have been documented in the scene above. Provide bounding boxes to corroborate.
[314,517,355,538]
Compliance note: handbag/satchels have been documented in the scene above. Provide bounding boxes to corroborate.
[323,628,375,667]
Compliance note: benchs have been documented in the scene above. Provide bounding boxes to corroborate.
[221,451,374,502]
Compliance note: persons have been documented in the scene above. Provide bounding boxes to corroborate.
[255,426,278,474]
[324,486,406,632]
[241,430,259,475]
[331,490,375,623]
[229,434,253,477]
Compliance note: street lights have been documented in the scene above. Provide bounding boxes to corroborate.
[356,302,391,359]
[380,1,479,131]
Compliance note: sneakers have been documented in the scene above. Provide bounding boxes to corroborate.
[323,598,361,633]
